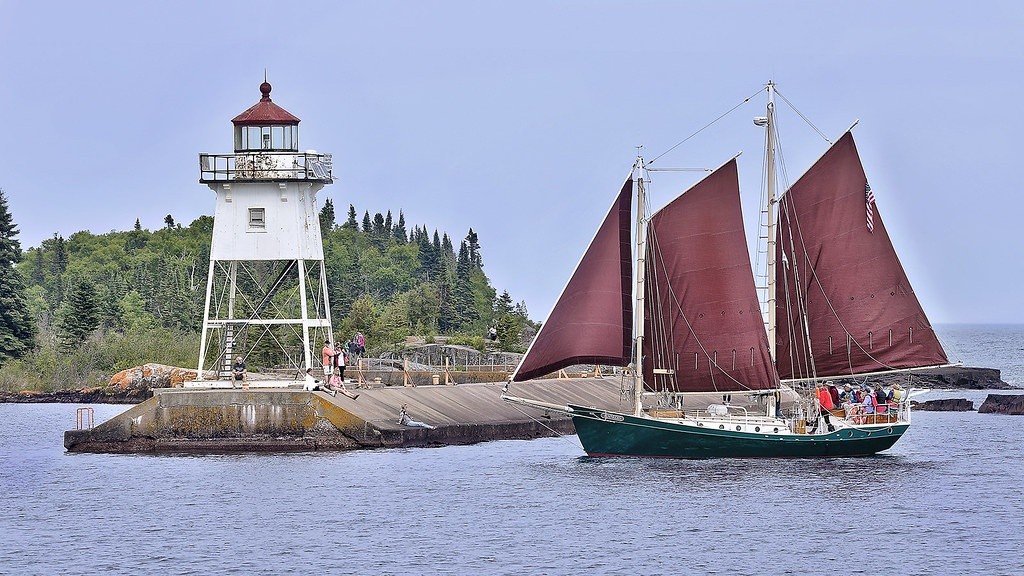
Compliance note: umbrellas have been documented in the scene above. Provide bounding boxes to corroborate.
[865,180,878,235]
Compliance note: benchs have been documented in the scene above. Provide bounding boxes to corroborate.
[650,410,685,419]
[866,412,897,424]
[831,409,844,417]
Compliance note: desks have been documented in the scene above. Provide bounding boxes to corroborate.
[842,402,861,419]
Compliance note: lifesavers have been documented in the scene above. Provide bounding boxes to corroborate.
[852,406,867,424]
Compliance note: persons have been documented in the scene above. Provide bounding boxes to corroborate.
[825,381,840,410]
[873,385,887,413]
[303,368,337,398]
[840,383,858,409]
[885,383,902,413]
[861,388,874,414]
[334,344,348,383]
[397,403,438,431]
[806,382,836,434]
[322,340,336,386]
[490,325,498,341]
[329,366,360,400]
[855,384,868,404]
[231,356,248,390]
[347,333,364,359]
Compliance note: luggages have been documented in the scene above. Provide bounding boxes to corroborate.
[807,431,815,434]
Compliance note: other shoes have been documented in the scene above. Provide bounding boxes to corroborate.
[353,395,360,400]
[332,389,337,398]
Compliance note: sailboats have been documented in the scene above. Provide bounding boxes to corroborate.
[498,79,963,459]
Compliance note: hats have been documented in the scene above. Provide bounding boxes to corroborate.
[324,341,330,344]
[843,384,851,388]
[825,381,835,387]
